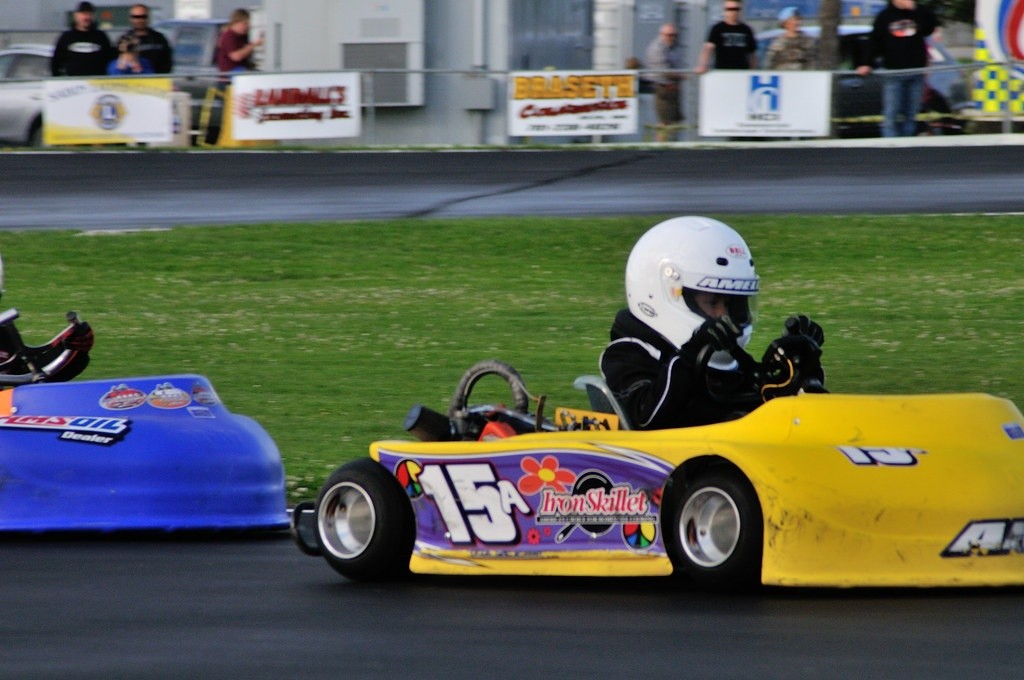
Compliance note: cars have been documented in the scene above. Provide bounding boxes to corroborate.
[152,19,224,127]
[752,26,967,129]
[0,41,60,149]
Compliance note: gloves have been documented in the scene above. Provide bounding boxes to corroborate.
[62,322,94,353]
[0,350,9,364]
[784,315,824,349]
[679,315,743,362]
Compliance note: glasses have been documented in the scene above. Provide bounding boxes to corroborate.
[658,31,678,39]
[129,15,147,18]
[723,7,742,11]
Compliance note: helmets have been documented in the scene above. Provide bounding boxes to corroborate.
[624,216,760,372]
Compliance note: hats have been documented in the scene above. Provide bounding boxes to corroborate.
[776,6,802,25]
[65,1,100,12]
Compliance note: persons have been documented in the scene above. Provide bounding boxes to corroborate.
[113,4,172,74]
[52,1,112,76]
[108,35,153,74]
[647,23,688,142]
[764,6,821,71]
[852,0,936,137]
[599,215,825,432]
[695,0,760,74]
[214,9,264,84]
